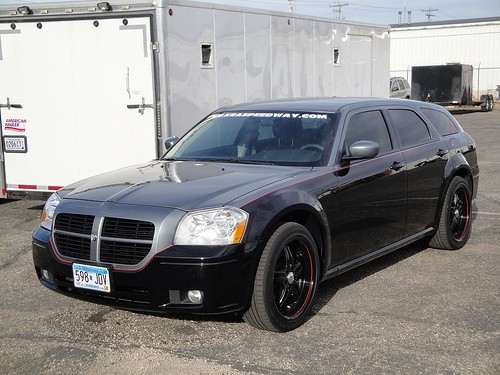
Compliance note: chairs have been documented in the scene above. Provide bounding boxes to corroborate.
[261,117,303,151]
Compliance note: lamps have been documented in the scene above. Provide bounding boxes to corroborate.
[96,2,112,11]
[17,6,33,15]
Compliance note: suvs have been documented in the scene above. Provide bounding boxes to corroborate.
[390,77,410,99]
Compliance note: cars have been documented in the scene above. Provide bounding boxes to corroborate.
[33,97,480,332]
